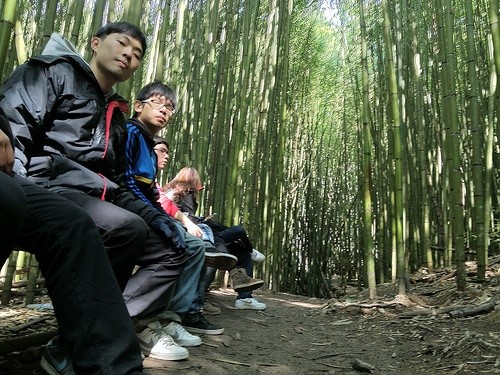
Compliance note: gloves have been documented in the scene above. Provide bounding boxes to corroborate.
[150,215,189,254]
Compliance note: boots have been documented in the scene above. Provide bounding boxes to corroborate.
[230,267,265,292]
[199,297,220,314]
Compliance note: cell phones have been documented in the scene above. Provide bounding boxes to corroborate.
[212,213,217,217]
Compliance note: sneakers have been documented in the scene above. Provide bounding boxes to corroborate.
[136,321,189,360]
[181,309,224,334]
[40,337,76,375]
[250,248,265,263]
[204,248,238,270]
[235,297,266,311]
[162,322,201,346]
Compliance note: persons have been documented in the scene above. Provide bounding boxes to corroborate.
[0,21,188,375]
[0,106,143,375]
[125,82,205,359]
[153,135,238,334]
[163,167,267,310]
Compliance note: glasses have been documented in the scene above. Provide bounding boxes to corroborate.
[154,148,168,156]
[140,97,175,115]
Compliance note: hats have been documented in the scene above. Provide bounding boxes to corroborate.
[152,135,169,149]
